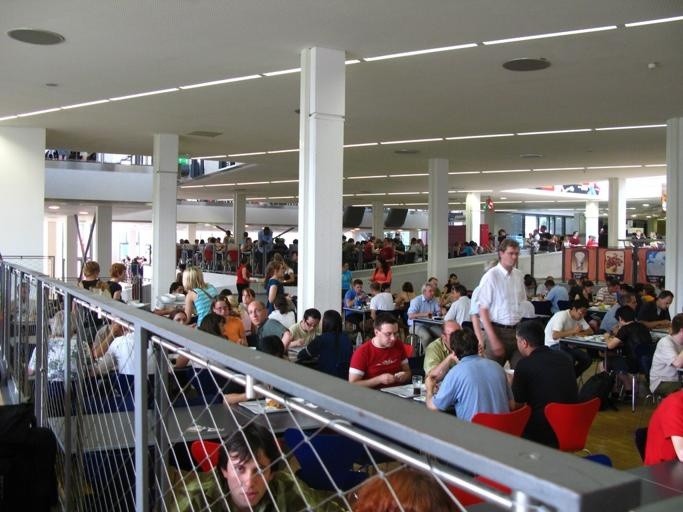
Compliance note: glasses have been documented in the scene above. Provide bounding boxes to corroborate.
[380,331,400,337]
[306,320,318,327]
[214,307,229,311]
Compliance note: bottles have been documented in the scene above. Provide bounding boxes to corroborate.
[356,331,363,349]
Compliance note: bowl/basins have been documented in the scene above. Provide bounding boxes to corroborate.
[173,292,185,301]
[161,293,176,302]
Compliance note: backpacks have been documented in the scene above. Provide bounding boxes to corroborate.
[577,372,619,412]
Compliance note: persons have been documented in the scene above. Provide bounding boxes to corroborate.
[526,224,599,253]
[425,329,515,423]
[604,304,652,403]
[72,261,112,327]
[348,312,411,389]
[151,282,184,318]
[256,335,284,360]
[90,323,158,374]
[342,228,427,271]
[427,277,441,302]
[596,279,619,304]
[599,295,636,335]
[343,279,371,329]
[369,283,394,320]
[177,230,236,268]
[369,254,392,292]
[544,301,594,379]
[544,280,569,314]
[606,275,613,286]
[118,256,148,283]
[568,279,582,306]
[640,290,674,322]
[424,320,483,385]
[342,262,353,301]
[536,276,553,298]
[263,262,284,312]
[288,308,321,344]
[248,301,290,353]
[638,285,658,319]
[643,389,683,466]
[106,263,126,300]
[225,295,252,336]
[407,282,443,352]
[239,227,298,285]
[170,422,336,512]
[219,289,232,296]
[635,282,644,295]
[298,310,353,380]
[524,274,537,298]
[236,256,256,304]
[448,226,507,259]
[28,310,94,381]
[10,282,37,320]
[200,313,226,339]
[91,320,128,358]
[471,260,517,356]
[650,313,683,395]
[626,228,666,253]
[619,283,633,305]
[395,282,417,329]
[479,238,527,368]
[511,320,578,449]
[182,266,218,328]
[441,284,471,329]
[575,281,594,308]
[442,273,460,296]
[170,309,187,325]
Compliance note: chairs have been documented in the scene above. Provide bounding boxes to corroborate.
[183,367,230,405]
[471,405,534,437]
[543,397,600,456]
[606,344,656,414]
[286,429,367,494]
[39,379,79,416]
[105,372,142,413]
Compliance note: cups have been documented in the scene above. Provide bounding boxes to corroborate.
[412,375,423,396]
[427,313,432,320]
